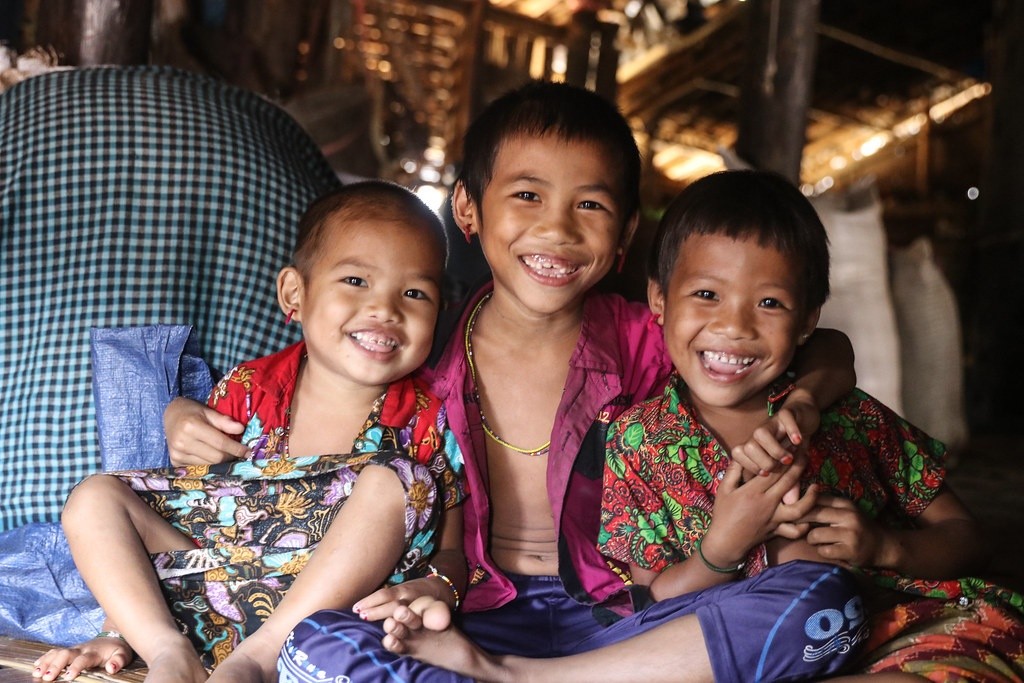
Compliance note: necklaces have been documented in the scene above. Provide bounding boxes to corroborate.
[465,292,551,456]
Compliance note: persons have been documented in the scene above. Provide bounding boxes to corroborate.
[164,78,870,682]
[30,180,467,681]
[596,169,1024,682]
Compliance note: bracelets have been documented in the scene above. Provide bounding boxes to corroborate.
[424,563,460,612]
[696,538,747,573]
